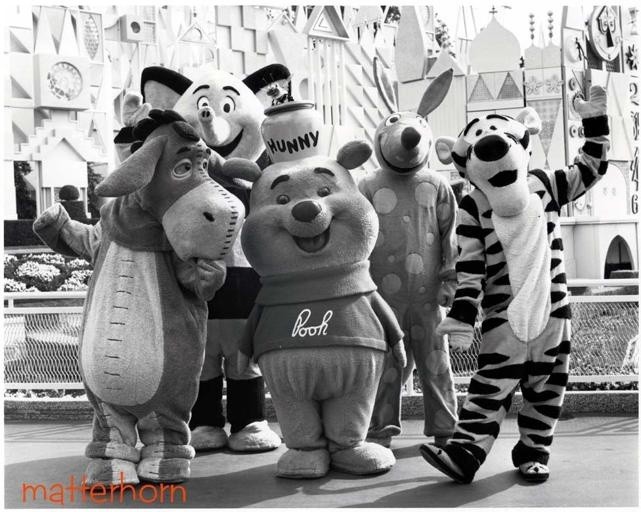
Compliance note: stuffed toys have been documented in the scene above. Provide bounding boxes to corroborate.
[221,96,409,481]
[420,83,612,485]
[140,61,293,454]
[29,106,246,492]
[355,55,458,449]
